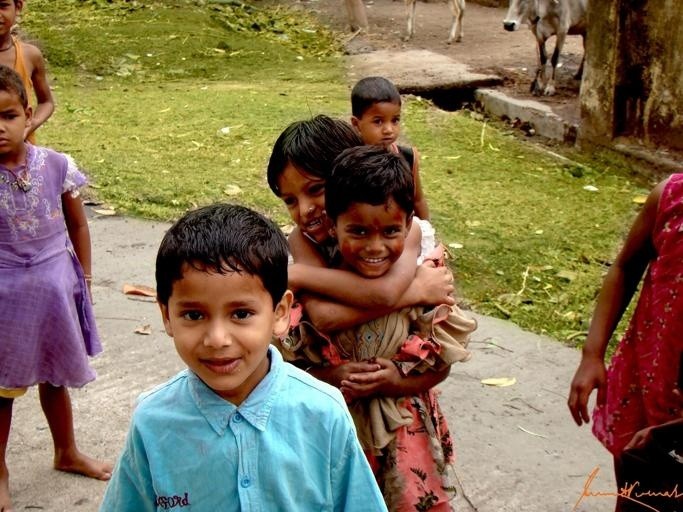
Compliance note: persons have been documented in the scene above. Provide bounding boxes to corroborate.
[101,204,390,512]
[350,76,429,222]
[268,144,476,511]
[566,171,682,511]
[0,0,54,145]
[0,66,117,511]
[267,115,456,334]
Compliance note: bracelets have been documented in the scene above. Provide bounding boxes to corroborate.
[83,273,91,282]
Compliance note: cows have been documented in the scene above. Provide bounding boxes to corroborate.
[502,0,589,99]
[402,0,466,46]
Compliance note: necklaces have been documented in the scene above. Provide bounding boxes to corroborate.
[0,143,30,193]
[0,38,12,54]
